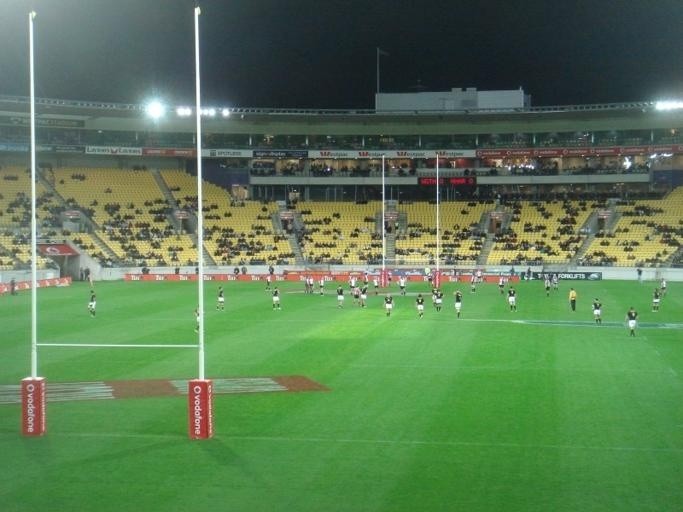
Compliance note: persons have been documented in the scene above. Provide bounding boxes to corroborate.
[1,133,683,326]
[193,304,199,334]
[624,307,639,337]
